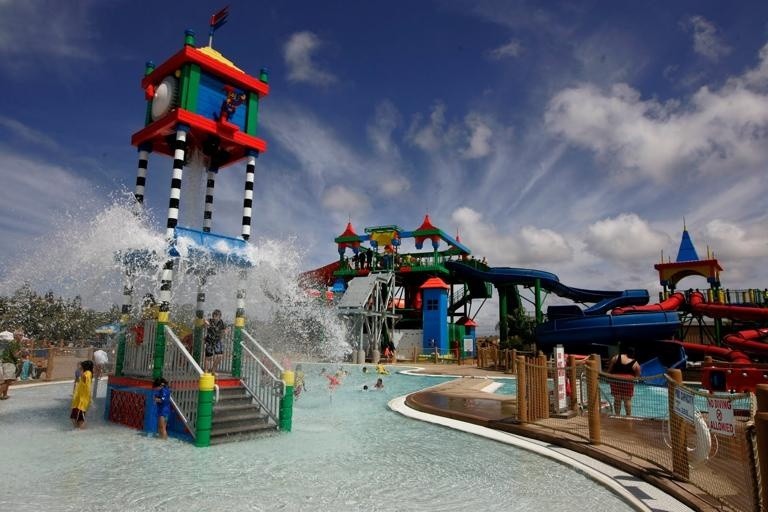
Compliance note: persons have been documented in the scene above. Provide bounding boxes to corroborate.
[358,248,373,269]
[153,378,170,440]
[384,346,394,361]
[362,367,371,375]
[763,288,768,303]
[141,293,159,320]
[373,378,384,391]
[204,309,230,376]
[293,365,306,400]
[399,253,428,266]
[94,349,108,381]
[606,343,640,416]
[318,367,349,378]
[376,362,390,375]
[442,255,462,261]
[470,256,487,265]
[0,330,36,399]
[70,360,94,430]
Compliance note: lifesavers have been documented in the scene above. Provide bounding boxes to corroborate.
[667,410,712,464]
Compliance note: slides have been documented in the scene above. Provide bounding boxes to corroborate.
[611,292,768,393]
[444,260,688,386]
